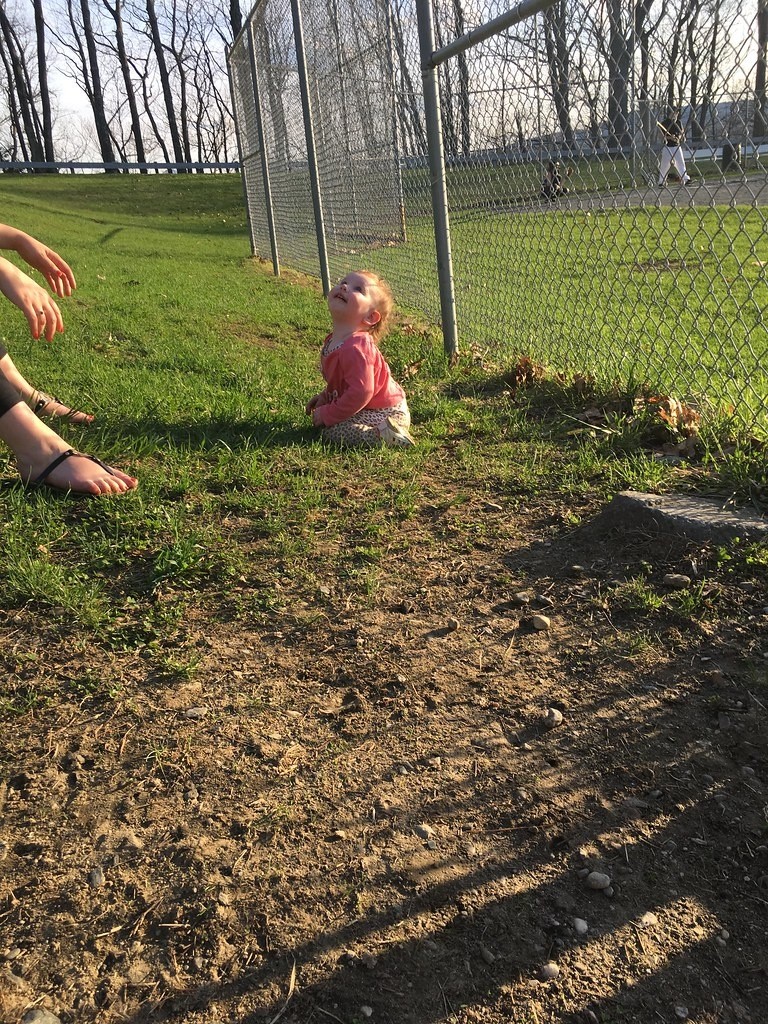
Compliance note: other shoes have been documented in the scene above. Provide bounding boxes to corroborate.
[376,417,416,448]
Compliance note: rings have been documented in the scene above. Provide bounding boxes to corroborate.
[37,311,46,316]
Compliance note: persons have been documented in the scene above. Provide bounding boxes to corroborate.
[541,160,573,205]
[657,106,695,187]
[304,271,413,450]
[0,226,141,498]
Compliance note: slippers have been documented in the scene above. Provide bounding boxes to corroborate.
[23,447,139,499]
[32,392,97,425]
